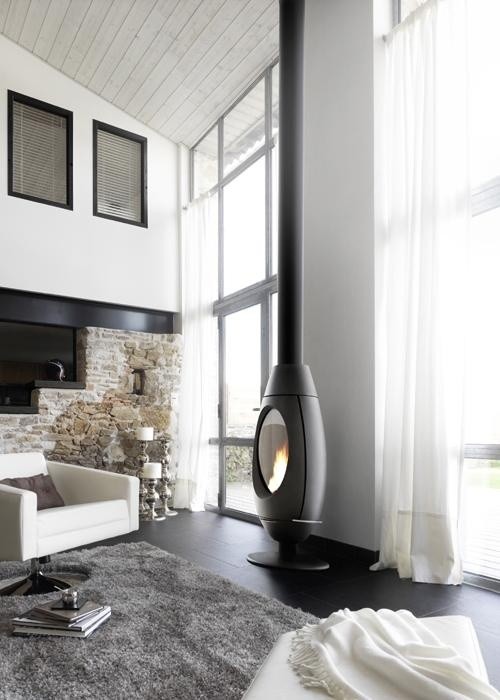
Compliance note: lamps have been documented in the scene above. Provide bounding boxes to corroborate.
[143,463,166,522]
[135,426,153,519]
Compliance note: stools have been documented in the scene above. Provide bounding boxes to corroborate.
[239,615,491,700]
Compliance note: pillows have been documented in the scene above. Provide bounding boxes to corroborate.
[1,475,65,512]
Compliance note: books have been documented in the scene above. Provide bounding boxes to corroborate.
[12,599,112,638]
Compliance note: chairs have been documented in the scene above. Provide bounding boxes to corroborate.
[0,450,140,563]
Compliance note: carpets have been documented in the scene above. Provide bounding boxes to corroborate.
[1,541,322,700]
